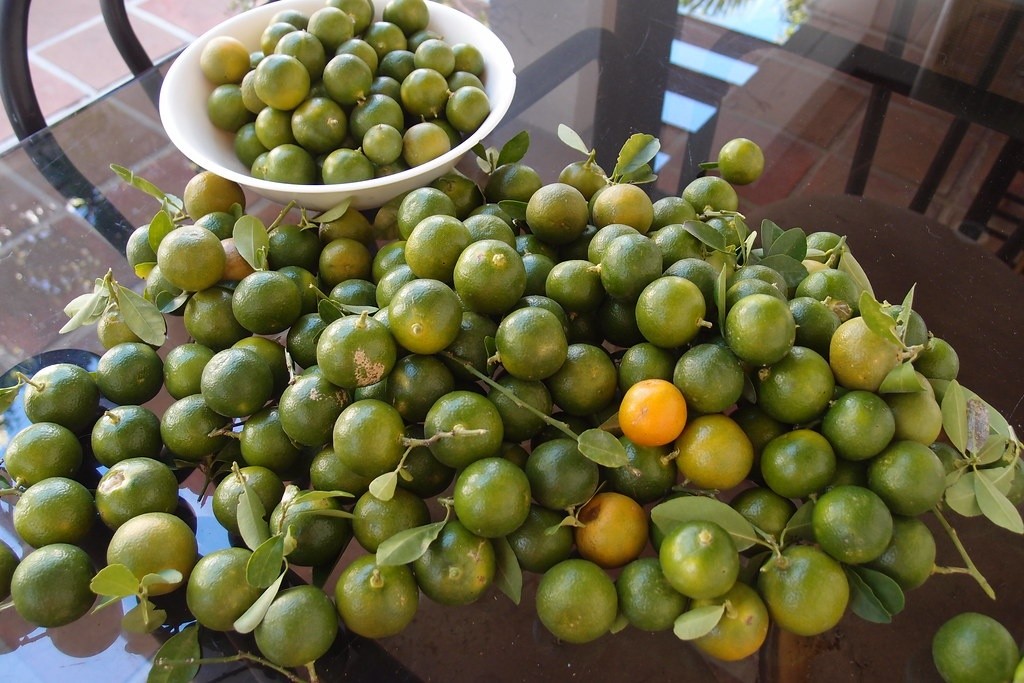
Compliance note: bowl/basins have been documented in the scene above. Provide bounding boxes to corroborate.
[159,0,516,214]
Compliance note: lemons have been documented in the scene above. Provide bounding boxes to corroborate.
[0,136,1023,682]
[201,0,491,185]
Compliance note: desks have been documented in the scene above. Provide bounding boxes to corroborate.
[0,1,1024,683]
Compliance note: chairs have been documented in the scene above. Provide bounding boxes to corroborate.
[735,1,1023,442]
